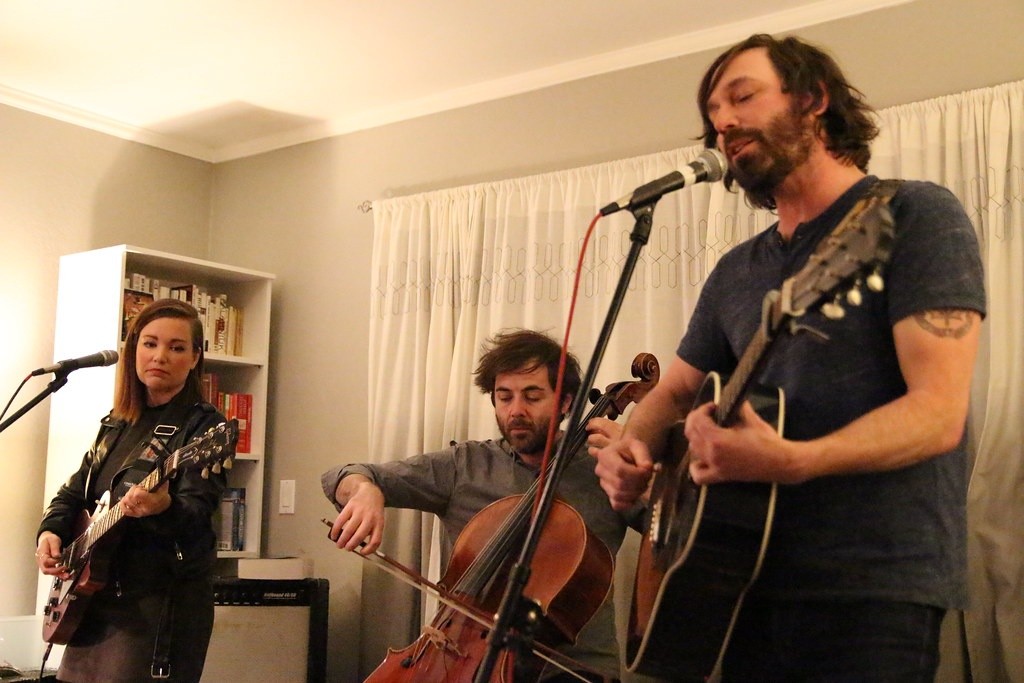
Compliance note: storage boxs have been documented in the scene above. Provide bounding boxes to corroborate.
[238,558,312,578]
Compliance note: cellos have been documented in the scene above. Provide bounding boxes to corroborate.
[360,352,662,683]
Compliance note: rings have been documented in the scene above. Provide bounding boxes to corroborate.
[134,501,140,507]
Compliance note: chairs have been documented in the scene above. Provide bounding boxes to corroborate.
[199,576,332,683]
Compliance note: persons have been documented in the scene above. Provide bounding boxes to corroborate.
[595,35,987,683]
[321,329,656,683]
[35,298,236,683]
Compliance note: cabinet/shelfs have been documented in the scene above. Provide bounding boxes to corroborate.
[1,244,276,672]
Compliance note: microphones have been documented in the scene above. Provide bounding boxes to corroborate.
[600,148,728,216]
[32,350,119,376]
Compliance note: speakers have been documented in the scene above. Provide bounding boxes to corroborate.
[195,578,330,683]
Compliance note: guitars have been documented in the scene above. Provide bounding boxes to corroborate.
[625,183,894,683]
[41,414,241,645]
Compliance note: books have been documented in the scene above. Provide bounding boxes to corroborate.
[200,372,252,453]
[212,488,246,551]
[123,273,244,356]
